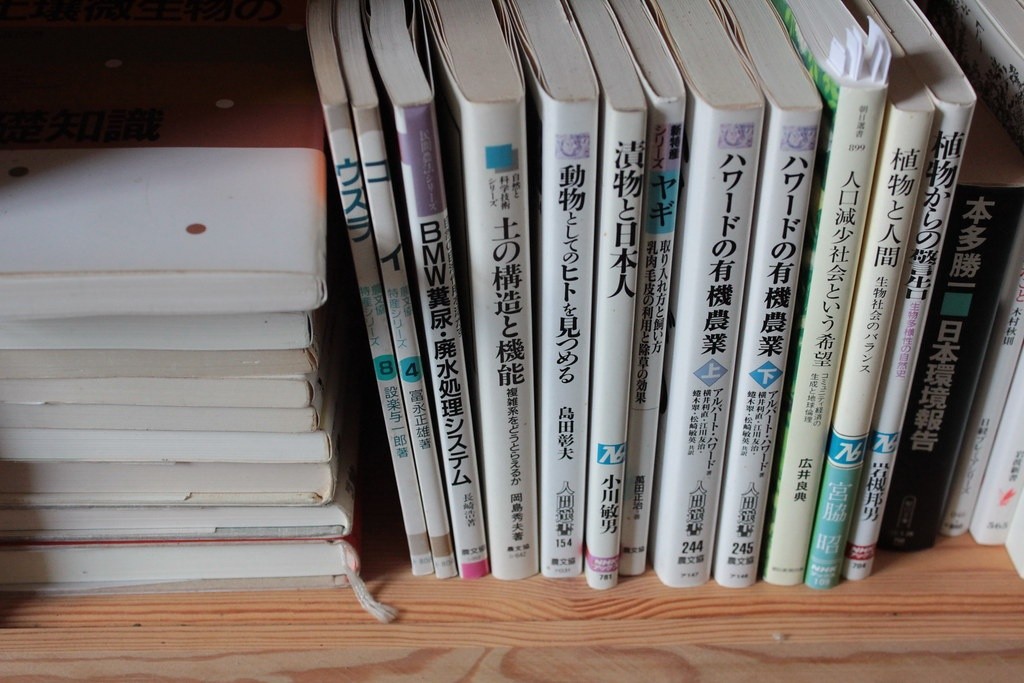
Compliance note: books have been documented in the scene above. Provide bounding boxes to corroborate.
[0,0,1024,595]
